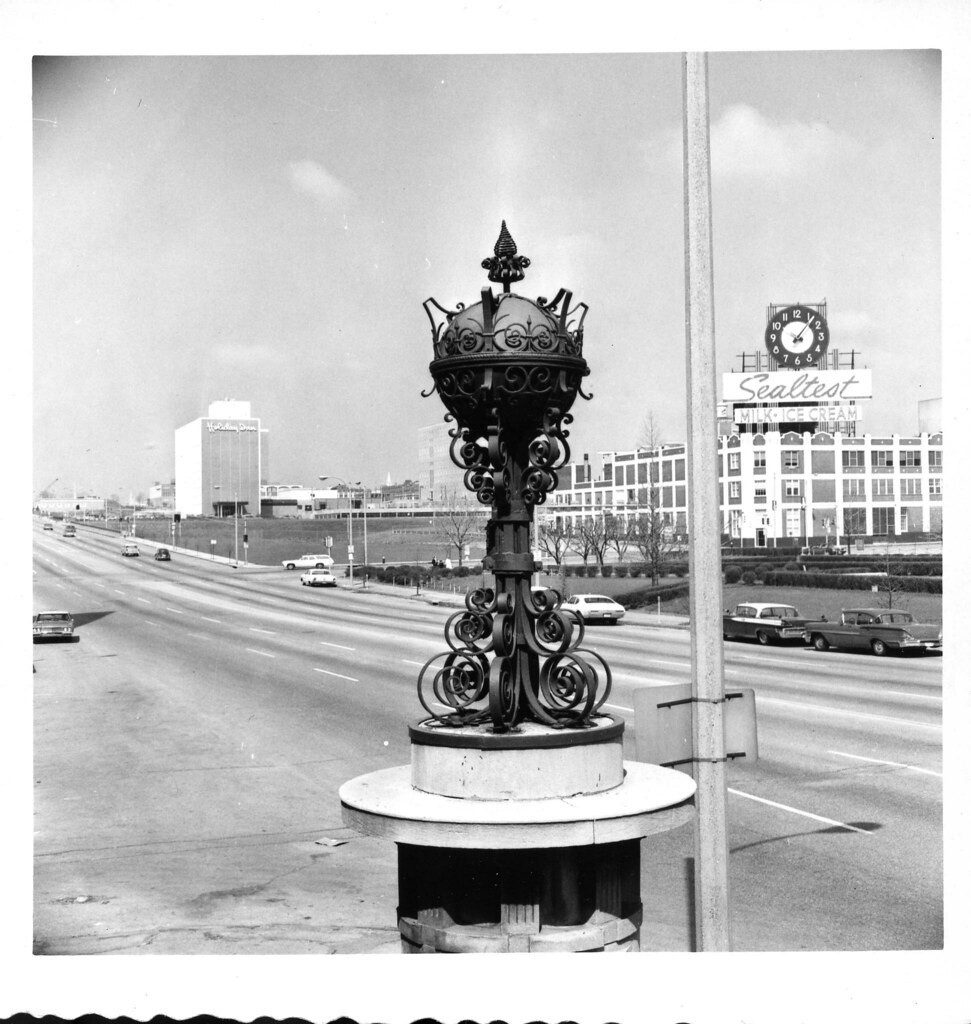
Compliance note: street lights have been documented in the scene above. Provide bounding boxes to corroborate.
[355,482,367,566]
[319,475,354,582]
[214,484,239,567]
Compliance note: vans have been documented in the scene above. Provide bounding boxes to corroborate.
[282,555,334,570]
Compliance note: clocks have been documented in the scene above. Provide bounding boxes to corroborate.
[765,307,828,369]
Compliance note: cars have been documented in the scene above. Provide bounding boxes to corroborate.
[804,606,941,654]
[723,603,824,645]
[33,611,74,640]
[118,544,141,556]
[154,548,171,560]
[554,594,627,623]
[65,525,77,537]
[300,569,337,587]
[41,523,55,530]
